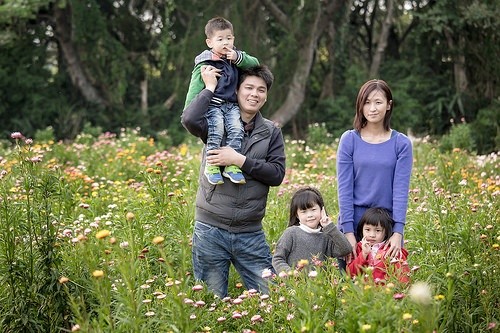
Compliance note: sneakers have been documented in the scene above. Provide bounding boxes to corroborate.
[222,164,246,184]
[204,163,225,185]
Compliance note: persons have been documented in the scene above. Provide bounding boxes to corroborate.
[184,16,258,184]
[272,187,352,278]
[336,79,413,274]
[181,64,286,298]
[346,206,410,293]
